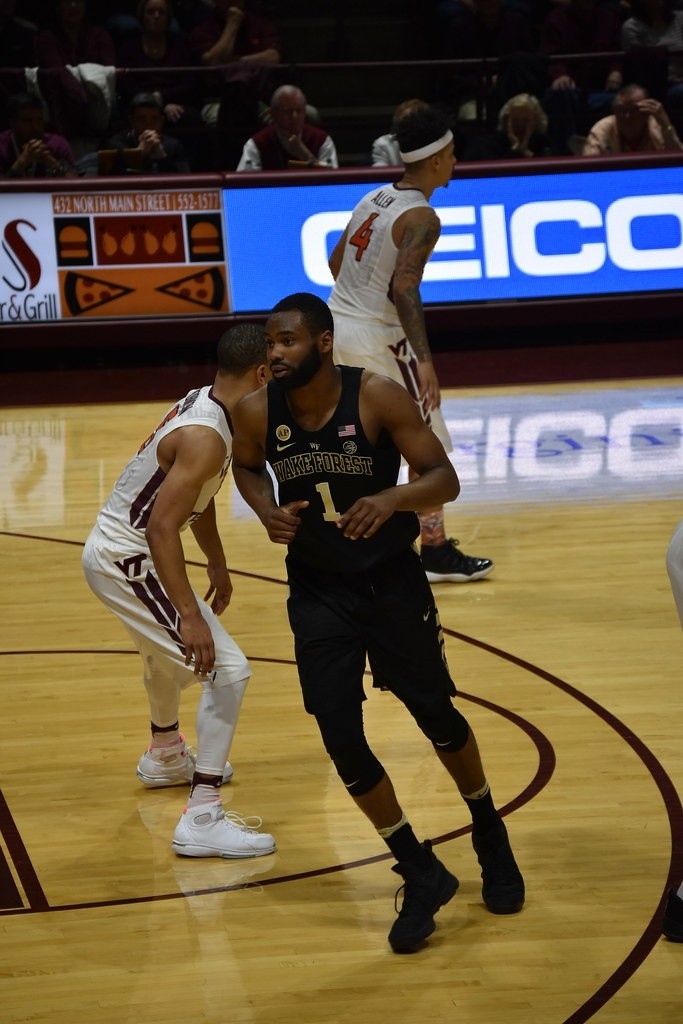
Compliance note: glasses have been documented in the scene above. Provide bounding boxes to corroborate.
[276,105,306,115]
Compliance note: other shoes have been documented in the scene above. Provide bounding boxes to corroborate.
[661,895,682,941]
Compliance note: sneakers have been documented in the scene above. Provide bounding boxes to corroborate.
[388,839,460,953]
[171,799,276,858]
[471,808,525,915]
[421,537,494,581]
[136,733,233,787]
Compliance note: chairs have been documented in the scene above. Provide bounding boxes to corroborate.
[179,127,259,171]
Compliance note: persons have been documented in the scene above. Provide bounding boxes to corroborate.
[84,325,273,860]
[663,526,683,942]
[325,99,495,584]
[1,0,683,178]
[232,292,526,953]
